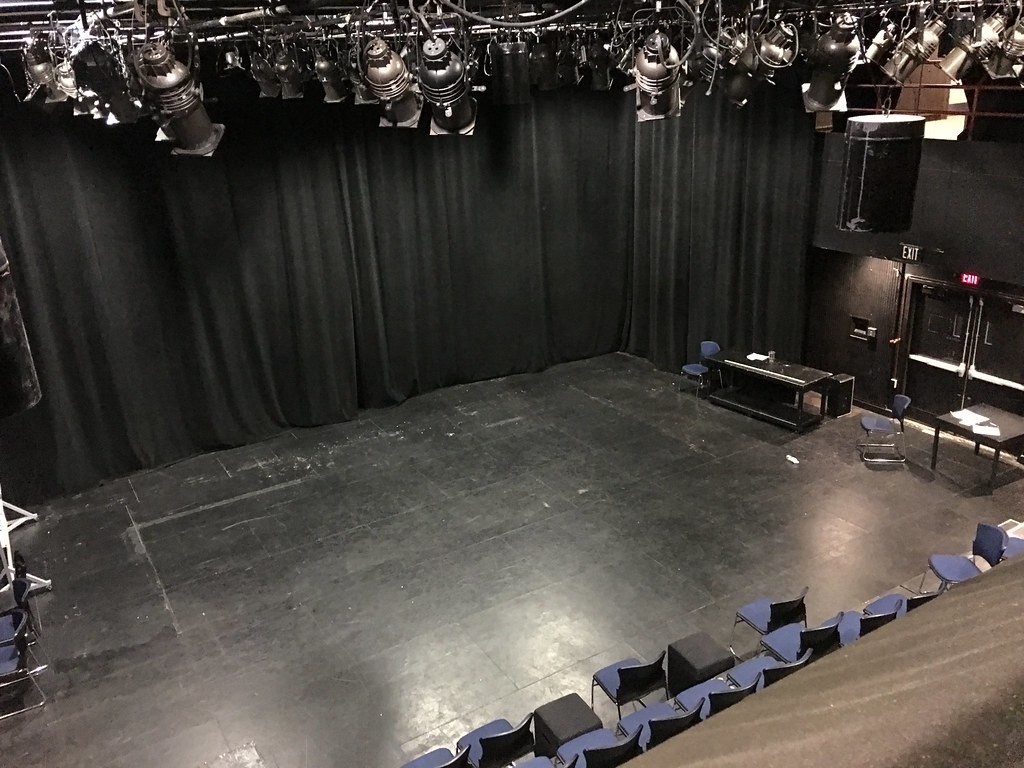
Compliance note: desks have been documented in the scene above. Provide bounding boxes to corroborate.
[667,630,736,698]
[931,402,1024,481]
[533,693,603,759]
[701,348,834,435]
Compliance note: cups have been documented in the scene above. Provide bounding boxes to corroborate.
[768,351,775,362]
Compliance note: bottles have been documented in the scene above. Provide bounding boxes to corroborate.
[786,454,800,464]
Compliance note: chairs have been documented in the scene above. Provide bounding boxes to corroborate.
[678,340,723,398]
[821,598,903,647]
[590,650,669,722]
[761,610,845,666]
[615,696,706,753]
[456,712,538,768]
[0,609,48,720]
[862,580,949,618]
[1000,537,1024,563]
[0,579,50,683]
[725,648,813,692]
[857,395,910,462]
[919,522,1009,595]
[729,585,809,661]
[556,723,644,768]
[400,744,471,768]
[673,672,761,722]
[513,753,579,768]
[0,550,49,647]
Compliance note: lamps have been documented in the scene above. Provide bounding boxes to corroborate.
[18,0,1024,160]
